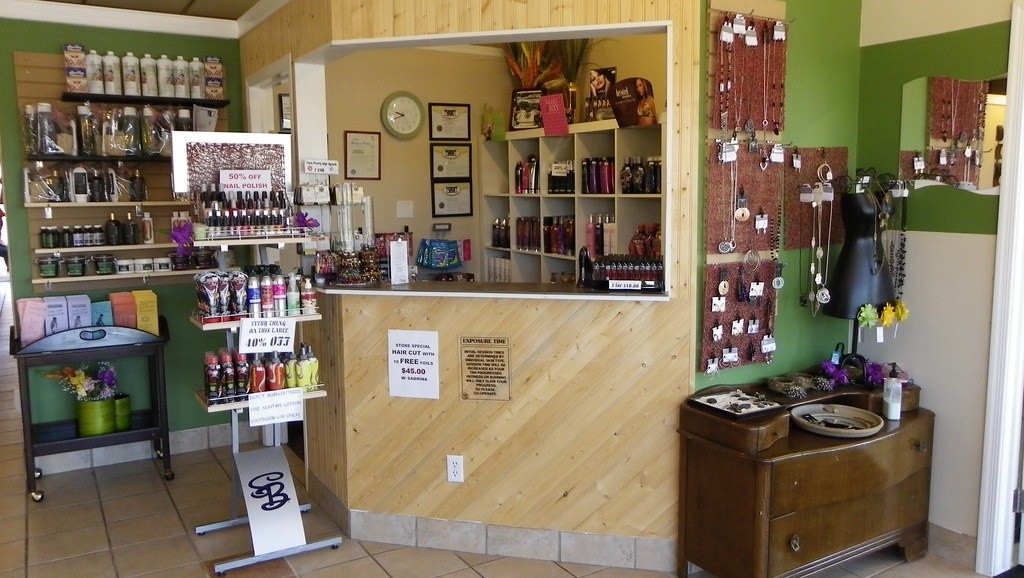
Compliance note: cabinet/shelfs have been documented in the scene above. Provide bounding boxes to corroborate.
[188,226,342,578]
[9,313,176,503]
[27,91,242,290]
[678,365,938,578]
[478,113,667,286]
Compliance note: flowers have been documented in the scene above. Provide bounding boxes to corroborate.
[858,303,879,329]
[881,302,896,328]
[57,361,122,402]
[895,300,908,322]
[866,363,886,385]
[821,358,849,386]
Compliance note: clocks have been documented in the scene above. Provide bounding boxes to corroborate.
[380,91,424,140]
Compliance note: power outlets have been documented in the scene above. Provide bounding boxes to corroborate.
[447,455,464,484]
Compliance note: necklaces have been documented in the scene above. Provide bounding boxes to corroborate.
[799,179,908,317]
[719,27,785,253]
[926,76,989,189]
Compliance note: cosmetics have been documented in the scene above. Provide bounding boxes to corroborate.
[193,264,316,316]
[24,50,206,278]
[200,182,293,235]
[205,348,320,405]
[488,149,662,292]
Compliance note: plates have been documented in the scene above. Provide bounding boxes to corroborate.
[791,403,884,438]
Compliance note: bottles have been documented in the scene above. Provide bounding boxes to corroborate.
[141,212,154,244]
[171,208,191,243]
[85,50,206,99]
[40,212,138,246]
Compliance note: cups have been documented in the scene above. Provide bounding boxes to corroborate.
[882,378,902,420]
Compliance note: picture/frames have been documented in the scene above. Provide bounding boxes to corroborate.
[509,87,547,131]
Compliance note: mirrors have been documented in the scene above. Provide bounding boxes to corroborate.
[899,72,1007,192]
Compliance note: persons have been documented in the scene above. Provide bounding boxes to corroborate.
[635,79,655,126]
[50,316,58,334]
[590,69,612,117]
[74,316,82,329]
[96,314,104,325]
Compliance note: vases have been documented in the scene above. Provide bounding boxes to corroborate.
[78,393,130,438]
